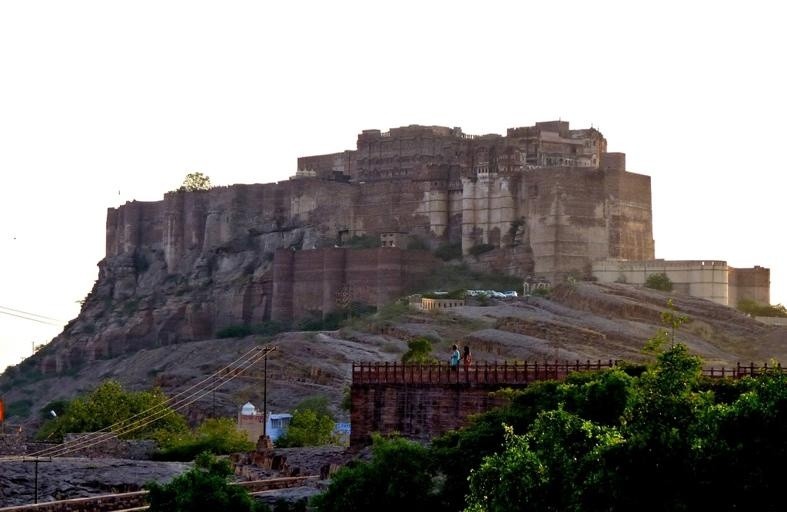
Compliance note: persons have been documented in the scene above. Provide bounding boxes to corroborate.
[446,343,472,382]
